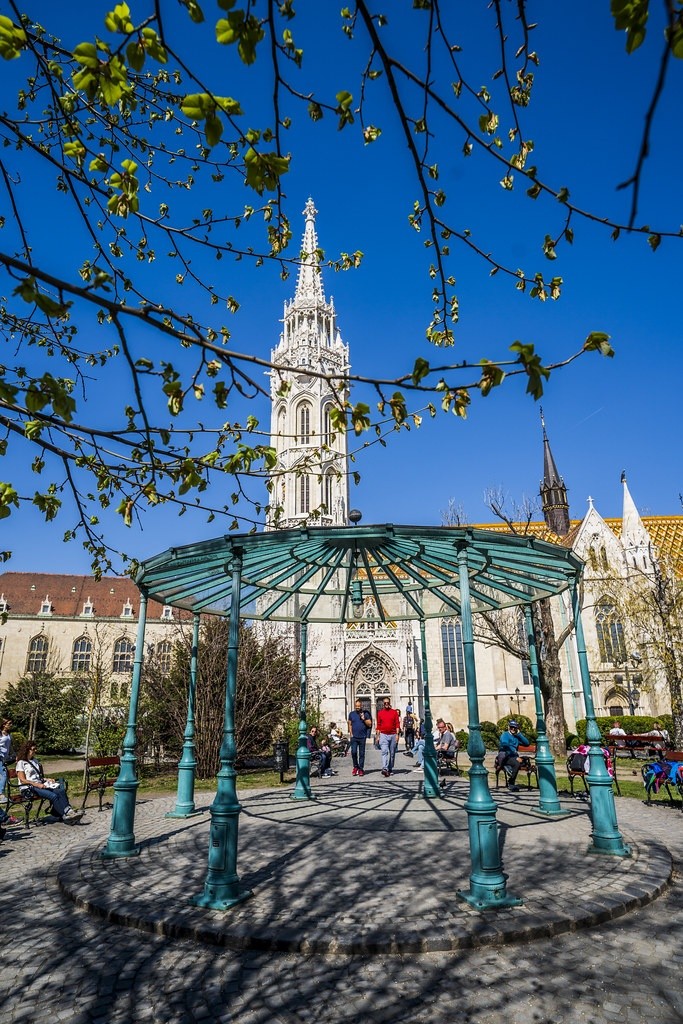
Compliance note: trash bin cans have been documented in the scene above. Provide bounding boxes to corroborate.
[271,739,290,783]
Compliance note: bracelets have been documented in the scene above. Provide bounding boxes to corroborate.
[363,719,367,721]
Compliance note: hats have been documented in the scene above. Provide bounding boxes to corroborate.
[510,721,517,727]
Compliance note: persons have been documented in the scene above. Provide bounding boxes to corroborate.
[16,741,83,822]
[375,697,403,776]
[330,722,351,753]
[307,726,337,778]
[609,722,627,747]
[499,722,530,791]
[403,701,457,773]
[632,722,670,759]
[0,717,13,804]
[348,700,372,775]
[572,738,613,778]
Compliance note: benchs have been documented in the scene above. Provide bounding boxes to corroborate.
[437,739,460,776]
[80,755,120,812]
[328,735,345,756]
[0,765,68,831]
[306,750,325,778]
[565,746,622,797]
[637,751,683,804]
[495,745,538,791]
[601,734,671,758]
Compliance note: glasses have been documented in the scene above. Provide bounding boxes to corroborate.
[311,730,317,733]
[384,702,390,705]
[30,747,37,751]
[438,727,443,728]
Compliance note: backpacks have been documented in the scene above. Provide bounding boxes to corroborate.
[570,753,586,772]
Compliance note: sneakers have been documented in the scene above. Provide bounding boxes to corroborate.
[353,767,358,776]
[358,768,364,776]
[1,815,25,830]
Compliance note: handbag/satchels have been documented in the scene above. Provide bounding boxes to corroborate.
[519,757,531,767]
[2,754,16,766]
[665,742,671,747]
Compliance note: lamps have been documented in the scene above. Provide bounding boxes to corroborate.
[350,540,365,617]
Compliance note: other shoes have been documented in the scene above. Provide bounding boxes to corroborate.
[508,784,519,791]
[388,769,394,775]
[0,794,8,803]
[403,752,413,758]
[413,762,421,767]
[63,809,85,825]
[321,772,330,778]
[381,769,389,777]
